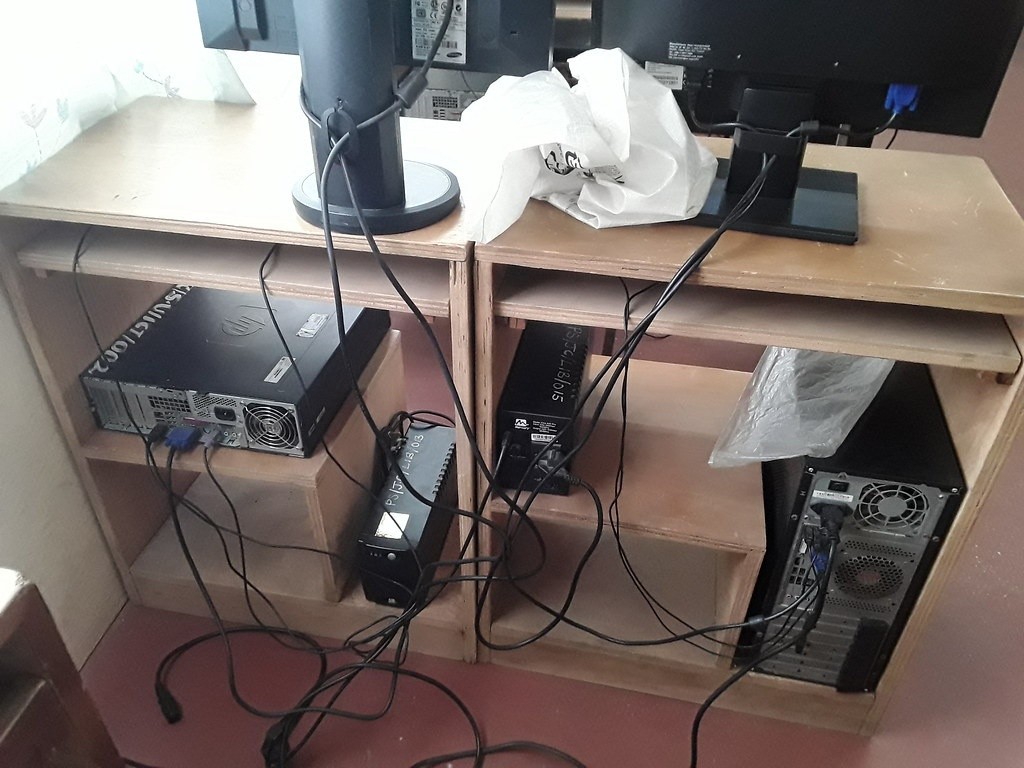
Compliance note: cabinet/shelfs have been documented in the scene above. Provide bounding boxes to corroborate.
[474,137,1024,739]
[0,96,473,664]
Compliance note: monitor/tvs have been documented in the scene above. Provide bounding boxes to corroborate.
[592,0,1024,246]
[196,0,557,76]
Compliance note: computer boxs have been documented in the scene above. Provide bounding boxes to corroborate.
[727,359,968,693]
[79,284,392,459]
